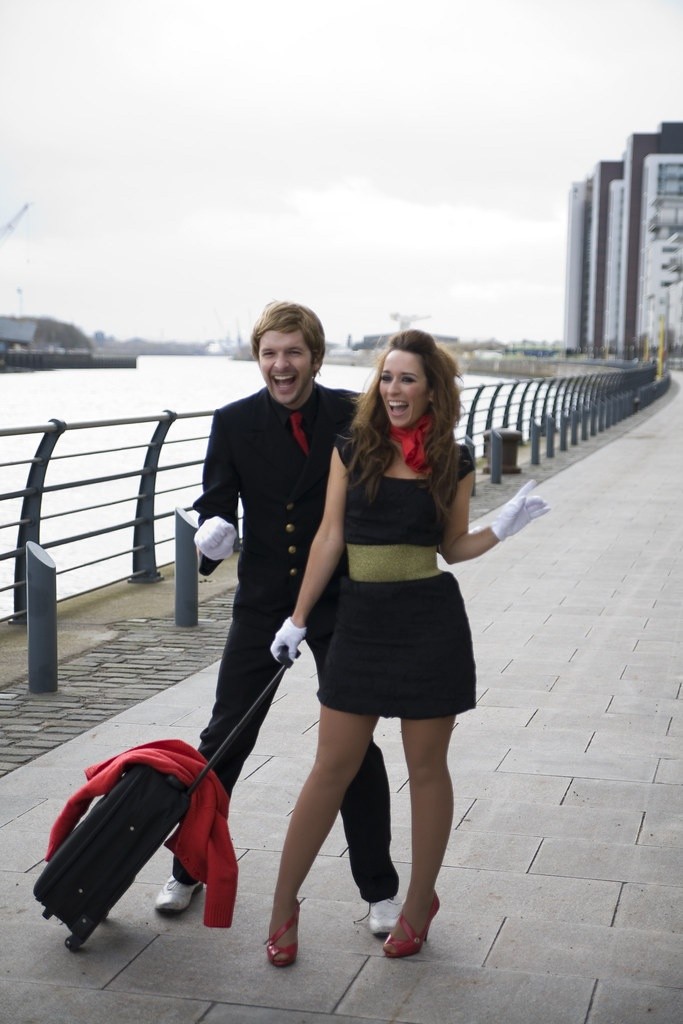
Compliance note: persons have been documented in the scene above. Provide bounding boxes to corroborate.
[155,303,407,936]
[265,329,552,965]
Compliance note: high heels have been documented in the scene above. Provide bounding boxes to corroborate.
[384,890,440,958]
[268,899,300,968]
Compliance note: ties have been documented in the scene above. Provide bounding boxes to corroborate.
[289,411,311,456]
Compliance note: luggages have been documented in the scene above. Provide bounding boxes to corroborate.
[32,649,301,950]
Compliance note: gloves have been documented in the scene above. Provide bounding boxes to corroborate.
[270,617,307,664]
[193,516,238,562]
[489,479,550,541]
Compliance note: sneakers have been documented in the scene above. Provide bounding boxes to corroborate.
[352,890,403,935]
[154,874,204,912]
